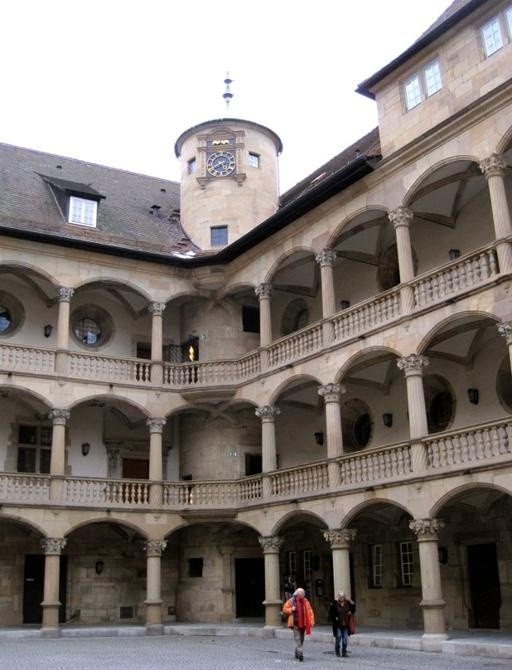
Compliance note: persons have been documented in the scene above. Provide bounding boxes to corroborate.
[281,587,316,661]
[327,591,357,657]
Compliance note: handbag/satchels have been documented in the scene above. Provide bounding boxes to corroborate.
[348,613,355,634]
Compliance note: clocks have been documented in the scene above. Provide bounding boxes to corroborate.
[206,151,237,178]
